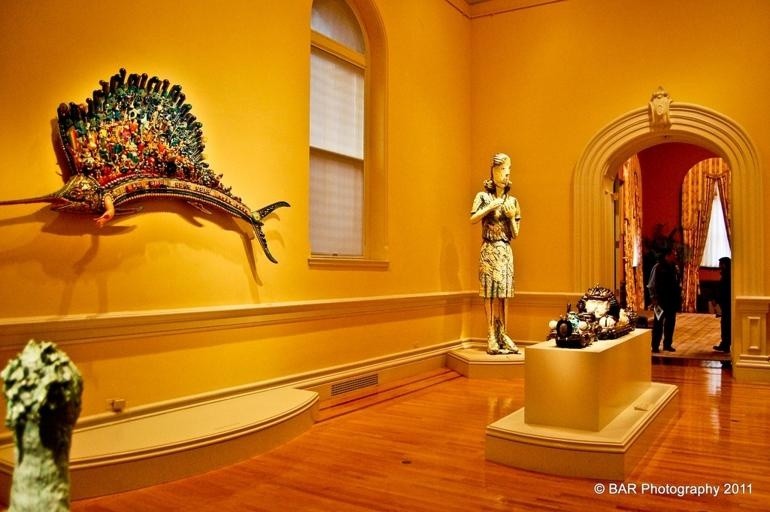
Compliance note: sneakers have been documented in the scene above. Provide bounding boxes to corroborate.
[713,343,730,352]
[652,347,660,353]
[662,344,676,352]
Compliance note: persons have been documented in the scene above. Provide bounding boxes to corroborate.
[646,246,681,355]
[718,257,736,345]
[471,153,521,354]
